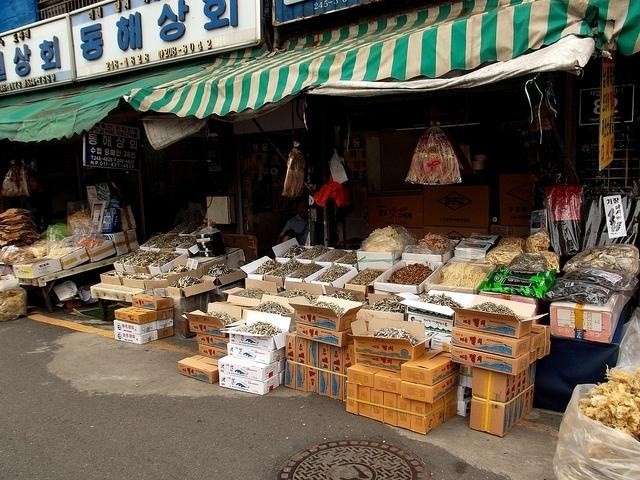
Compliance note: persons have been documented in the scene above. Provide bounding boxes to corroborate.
[281,204,309,245]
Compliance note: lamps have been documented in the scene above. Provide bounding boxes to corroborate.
[549,288,623,345]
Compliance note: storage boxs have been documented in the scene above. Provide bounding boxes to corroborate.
[242,294,294,331]
[449,326,530,359]
[318,370,330,398]
[67,284,98,308]
[295,362,306,391]
[114,320,157,335]
[399,373,457,406]
[374,259,442,295]
[287,261,331,283]
[87,237,117,263]
[272,238,313,259]
[140,222,207,257]
[12,257,62,279]
[177,355,219,385]
[287,295,364,331]
[306,366,318,394]
[240,256,289,276]
[383,393,400,427]
[499,173,537,226]
[368,193,422,228]
[168,275,216,300]
[278,358,286,372]
[423,185,490,228]
[265,258,310,282]
[423,228,490,237]
[402,239,460,267]
[275,280,323,307]
[490,224,530,239]
[227,343,285,364]
[184,302,242,338]
[114,306,157,324]
[529,324,551,365]
[335,249,357,264]
[410,385,459,437]
[448,294,551,338]
[331,346,343,372]
[201,267,247,286]
[157,319,175,330]
[218,356,278,382]
[330,372,343,402]
[45,246,90,270]
[295,337,306,362]
[349,318,438,361]
[158,308,174,320]
[285,360,295,390]
[315,248,352,263]
[345,381,358,415]
[278,372,285,385]
[218,374,280,395]
[200,247,246,272]
[342,345,355,376]
[131,294,175,311]
[307,264,358,285]
[345,268,385,293]
[396,290,476,332]
[357,385,370,419]
[222,278,277,308]
[397,395,411,432]
[449,345,530,376]
[158,326,174,340]
[356,293,407,321]
[346,362,381,388]
[296,323,354,347]
[220,311,291,350]
[370,389,383,424]
[358,238,418,265]
[322,283,366,302]
[318,343,331,368]
[197,333,229,349]
[374,368,400,394]
[114,330,158,345]
[198,344,227,359]
[103,229,140,256]
[426,256,493,308]
[101,249,199,290]
[295,245,334,260]
[399,350,458,386]
[471,362,541,403]
[284,335,296,361]
[305,340,317,368]
[120,205,136,232]
[469,383,535,439]
